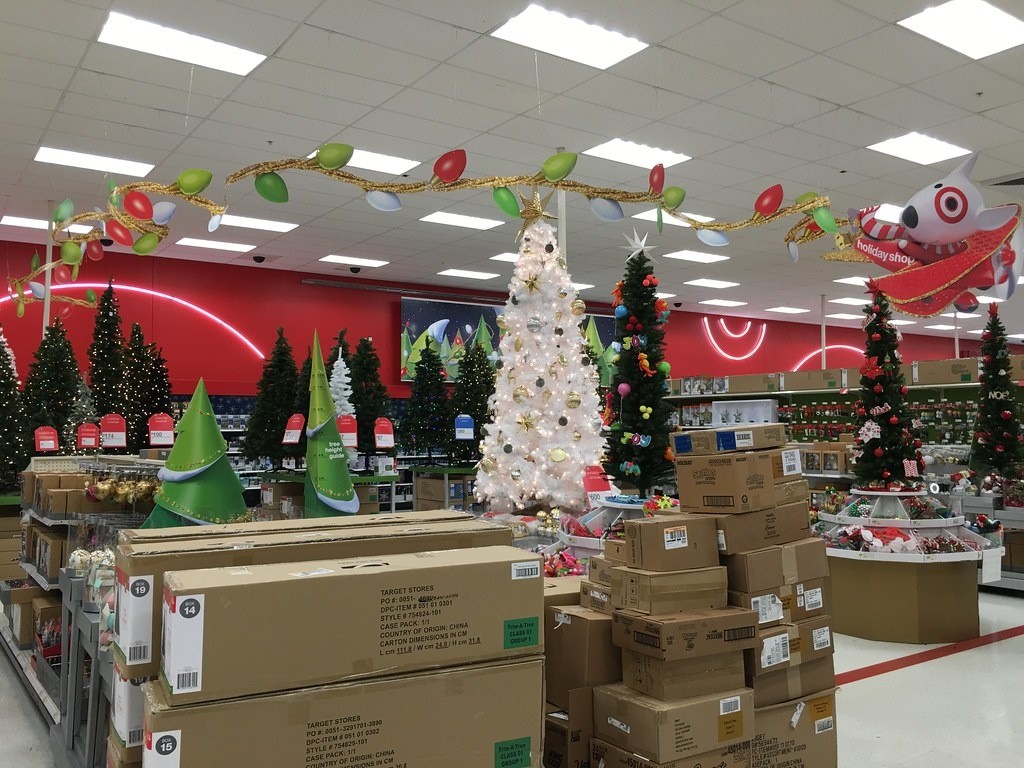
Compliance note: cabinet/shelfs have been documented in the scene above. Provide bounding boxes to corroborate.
[169,427,482,514]
[510,494,682,579]
[802,469,1024,645]
[663,383,984,449]
[0,507,142,728]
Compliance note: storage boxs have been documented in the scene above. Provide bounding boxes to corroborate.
[545,423,838,767]
[665,355,1024,396]
[254,455,399,521]
[106,506,546,768]
[0,471,139,650]
[413,475,491,516]
[799,433,858,513]
[668,403,712,426]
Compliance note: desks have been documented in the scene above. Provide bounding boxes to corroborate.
[408,465,494,516]
[257,470,401,513]
